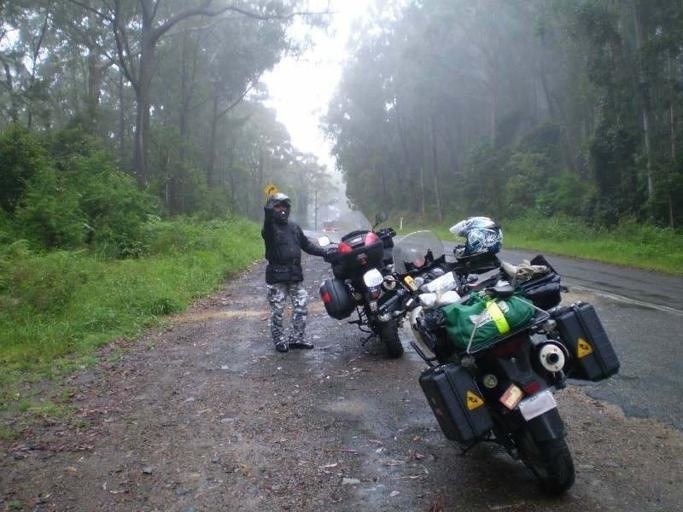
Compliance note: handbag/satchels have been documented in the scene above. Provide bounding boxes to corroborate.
[441,285,535,350]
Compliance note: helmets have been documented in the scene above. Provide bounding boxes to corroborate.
[448,217,503,261]
[269,192,292,224]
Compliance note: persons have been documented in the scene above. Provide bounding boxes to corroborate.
[261,185,338,352]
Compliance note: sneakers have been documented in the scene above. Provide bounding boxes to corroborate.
[290,341,314,349]
[276,343,288,352]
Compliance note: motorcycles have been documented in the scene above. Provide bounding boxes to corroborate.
[316,229,404,356]
[378,230,620,493]
